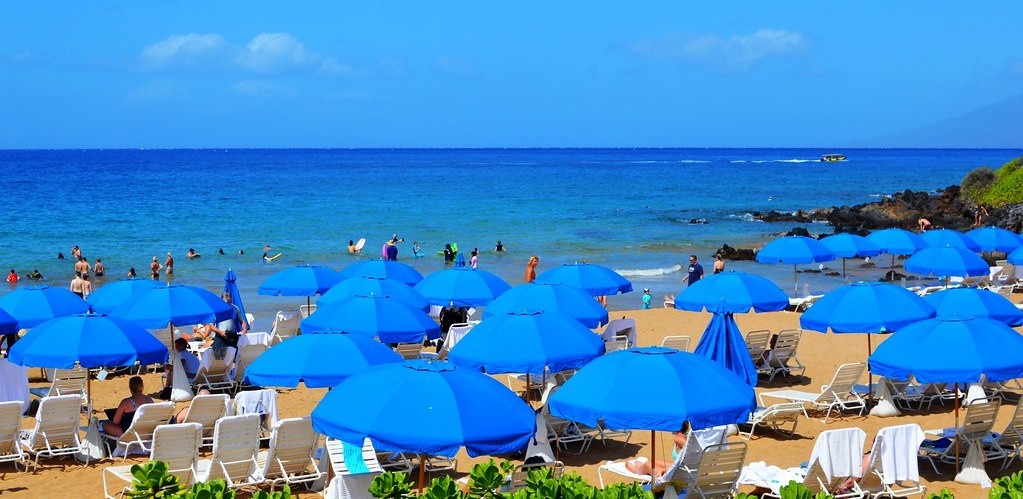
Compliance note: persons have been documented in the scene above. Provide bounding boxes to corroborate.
[642,288,652,309]
[127,247,200,280]
[347,234,507,264]
[755,334,778,366]
[525,256,540,283]
[597,295,607,307]
[626,420,690,477]
[834,436,875,495]
[683,254,727,286]
[6,245,105,300]
[918,218,933,230]
[100,292,248,438]
[219,243,271,262]
[975,203,990,226]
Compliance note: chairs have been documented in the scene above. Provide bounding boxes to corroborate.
[0,259,1023,499]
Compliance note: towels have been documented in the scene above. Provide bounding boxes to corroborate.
[447,325,476,351]
[601,319,637,348]
[989,265,1004,281]
[735,427,867,495]
[864,423,926,485]
[230,387,281,433]
[604,423,729,482]
[237,332,273,347]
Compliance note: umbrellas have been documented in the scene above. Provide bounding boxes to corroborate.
[7,311,168,426]
[549,345,757,494]
[481,258,633,329]
[755,227,1023,475]
[220,268,251,332]
[299,257,442,343]
[258,263,348,316]
[243,328,404,391]
[85,274,166,316]
[0,285,92,336]
[108,281,237,358]
[674,269,790,388]
[311,356,538,496]
[413,266,512,308]
[445,307,605,404]
[455,251,466,268]
